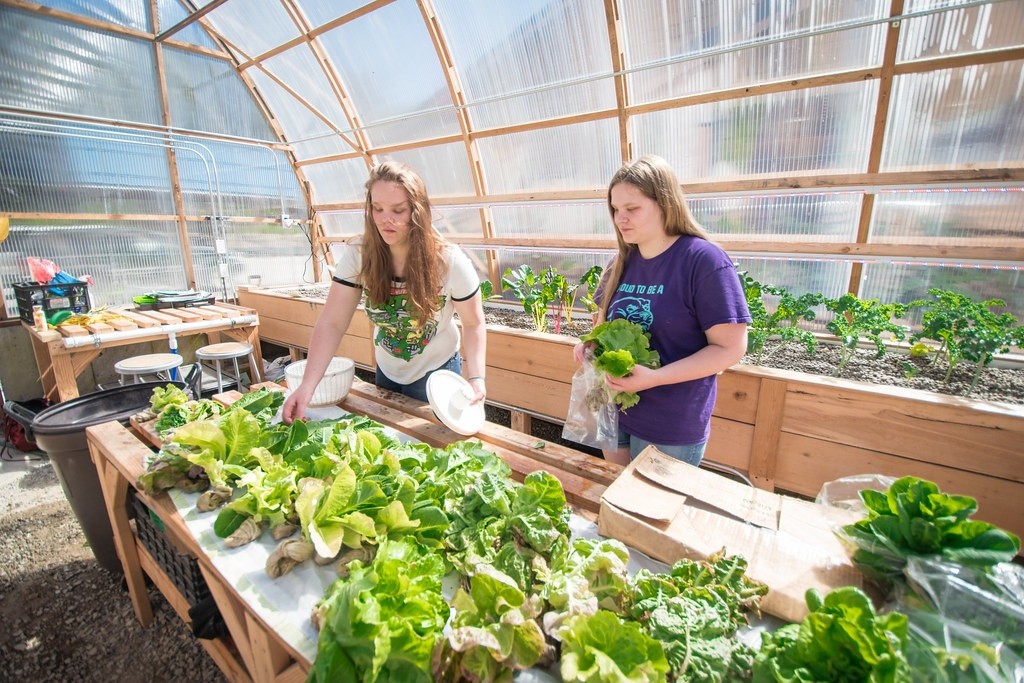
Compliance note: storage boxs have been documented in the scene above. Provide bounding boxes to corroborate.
[125,488,211,606]
[12,281,91,324]
[598,444,886,625]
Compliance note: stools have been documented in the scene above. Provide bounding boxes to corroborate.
[115,353,184,386]
[196,341,261,399]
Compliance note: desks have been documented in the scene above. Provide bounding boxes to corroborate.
[86,374,626,683]
[21,301,266,404]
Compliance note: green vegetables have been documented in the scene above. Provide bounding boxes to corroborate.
[579,318,660,414]
[134,385,1024,683]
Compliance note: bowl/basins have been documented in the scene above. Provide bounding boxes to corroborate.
[283,356,357,407]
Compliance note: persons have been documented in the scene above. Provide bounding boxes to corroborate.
[282,161,486,424]
[573,155,752,469]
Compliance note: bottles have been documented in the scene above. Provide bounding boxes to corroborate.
[33,304,47,331]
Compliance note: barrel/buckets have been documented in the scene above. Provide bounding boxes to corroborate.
[2,363,202,575]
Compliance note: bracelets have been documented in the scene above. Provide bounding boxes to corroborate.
[468,374,485,382]
